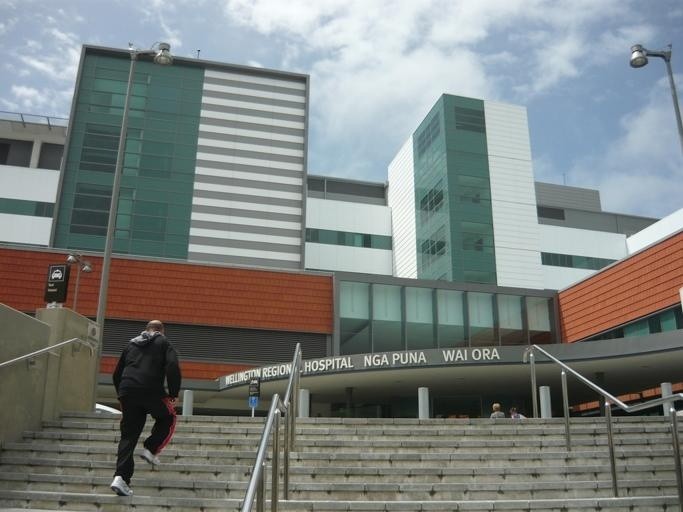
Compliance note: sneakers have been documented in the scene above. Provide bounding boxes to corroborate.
[109,476,134,496]
[141,450,161,467]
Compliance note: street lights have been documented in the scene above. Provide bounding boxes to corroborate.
[95,39,174,324]
[628,38,683,159]
[66,251,92,312]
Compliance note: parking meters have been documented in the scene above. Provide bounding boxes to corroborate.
[249,376,261,418]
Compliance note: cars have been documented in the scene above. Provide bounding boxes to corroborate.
[96,403,121,414]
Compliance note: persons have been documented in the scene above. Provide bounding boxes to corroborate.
[109,320,182,497]
[509,407,527,419]
[489,403,505,418]
